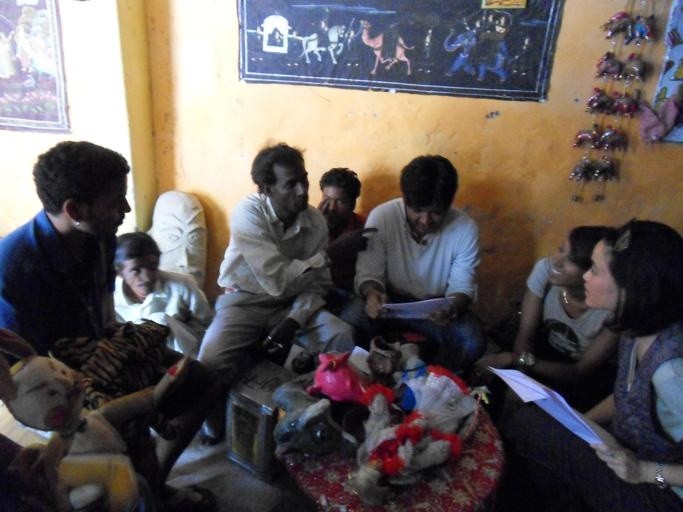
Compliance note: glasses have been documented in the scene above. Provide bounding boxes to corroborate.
[613,217,636,253]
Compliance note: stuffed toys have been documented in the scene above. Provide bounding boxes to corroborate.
[278,334,485,504]
[0,328,185,512]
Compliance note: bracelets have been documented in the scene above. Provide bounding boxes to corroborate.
[654,461,668,492]
[516,348,534,372]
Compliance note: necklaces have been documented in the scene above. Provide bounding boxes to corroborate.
[560,288,587,311]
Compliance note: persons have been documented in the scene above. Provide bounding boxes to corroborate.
[456,226,615,424]
[333,155,484,367]
[495,218,682,512]
[194,145,354,450]
[0,141,223,512]
[150,190,206,290]
[107,231,214,361]
[313,168,363,317]
[0,3,60,124]
